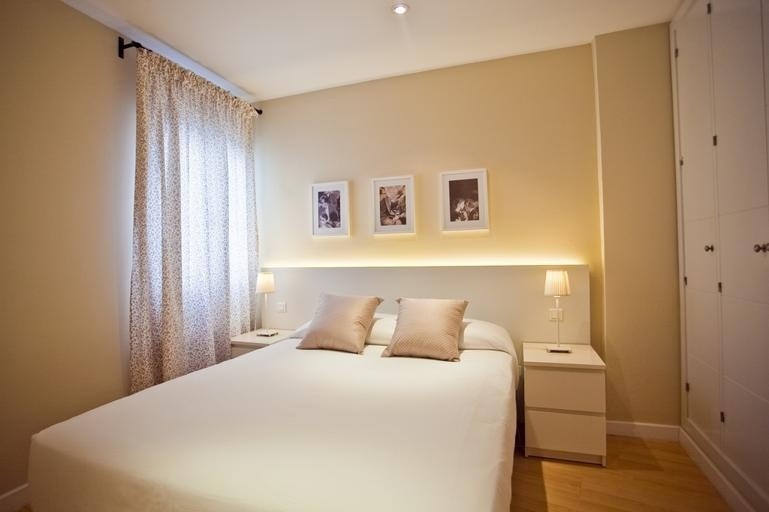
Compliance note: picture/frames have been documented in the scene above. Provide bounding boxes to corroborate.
[371,174,417,236]
[309,179,351,240]
[440,167,490,234]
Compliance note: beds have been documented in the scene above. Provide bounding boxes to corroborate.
[26,311,520,511]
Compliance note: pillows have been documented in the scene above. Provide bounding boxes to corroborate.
[379,295,470,363]
[294,289,386,356]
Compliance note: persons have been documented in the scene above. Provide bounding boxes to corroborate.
[392,194,406,225]
[464,199,479,220]
[317,195,330,222]
[380,187,401,225]
[451,198,469,222]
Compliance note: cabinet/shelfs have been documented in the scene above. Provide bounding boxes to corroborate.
[666,0,769,512]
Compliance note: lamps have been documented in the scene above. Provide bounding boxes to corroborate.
[254,271,280,338]
[543,269,574,355]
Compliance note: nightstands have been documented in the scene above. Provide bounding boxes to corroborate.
[522,339,609,469]
[228,328,296,362]
